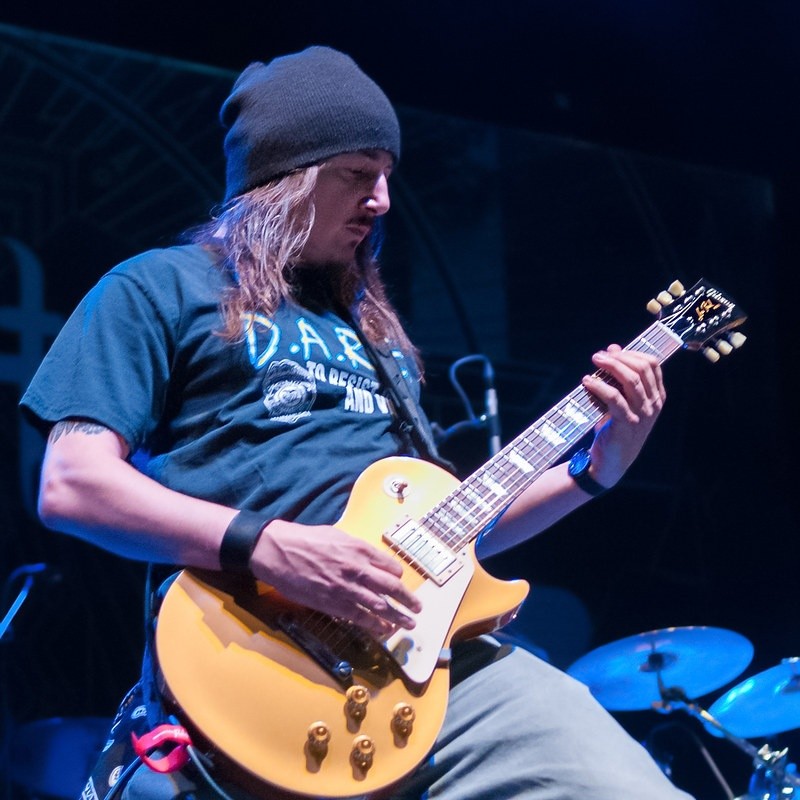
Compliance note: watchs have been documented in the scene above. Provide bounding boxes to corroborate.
[568,447,608,498]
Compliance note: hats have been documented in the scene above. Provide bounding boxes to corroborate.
[219,45,405,202]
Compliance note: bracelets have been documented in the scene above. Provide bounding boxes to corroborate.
[219,508,280,575]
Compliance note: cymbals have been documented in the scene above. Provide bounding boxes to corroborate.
[563,623,755,714]
[703,656,800,741]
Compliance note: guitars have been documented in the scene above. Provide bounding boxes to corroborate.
[149,276,751,799]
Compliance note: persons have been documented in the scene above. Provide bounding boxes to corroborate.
[19,44,700,800]
